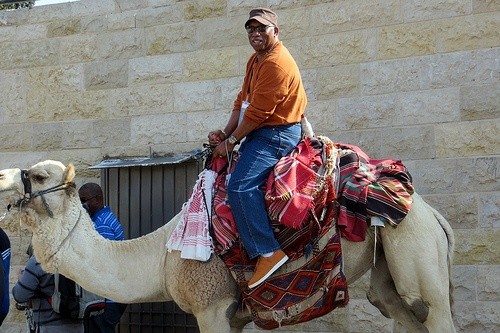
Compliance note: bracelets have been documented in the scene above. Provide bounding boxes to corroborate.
[219,127,228,139]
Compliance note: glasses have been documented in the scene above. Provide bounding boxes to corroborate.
[79,197,97,204]
[244,24,278,34]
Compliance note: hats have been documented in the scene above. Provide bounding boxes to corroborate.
[244,9,278,27]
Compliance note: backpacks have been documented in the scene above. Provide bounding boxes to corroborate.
[36,273,106,322]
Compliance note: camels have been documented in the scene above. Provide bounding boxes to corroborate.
[0,158,456,333]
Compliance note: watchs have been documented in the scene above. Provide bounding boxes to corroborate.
[229,136,240,145]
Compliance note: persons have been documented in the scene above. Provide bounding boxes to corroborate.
[11,246,84,333]
[0,227,11,326]
[77,182,128,333]
[207,7,308,289]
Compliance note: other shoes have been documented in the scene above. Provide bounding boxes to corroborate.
[246,248,290,290]
